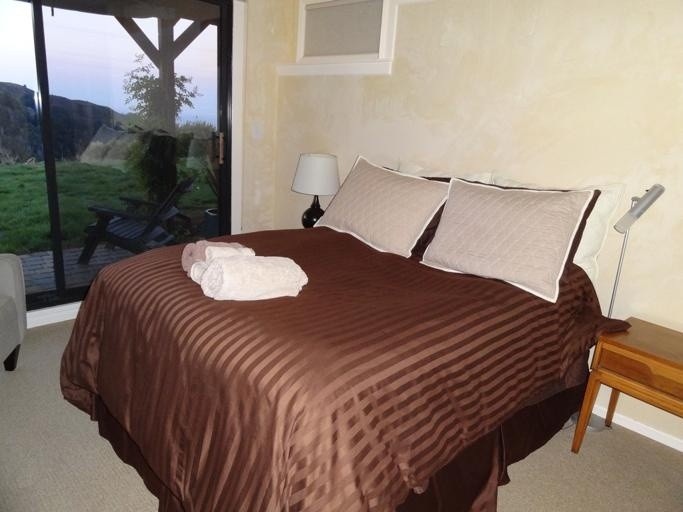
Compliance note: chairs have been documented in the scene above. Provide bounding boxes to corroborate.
[77,170,201,269]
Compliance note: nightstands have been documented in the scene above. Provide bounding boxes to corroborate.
[568,316,682,455]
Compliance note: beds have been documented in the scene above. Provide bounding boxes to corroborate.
[84,156,607,512]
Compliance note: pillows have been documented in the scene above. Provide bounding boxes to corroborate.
[312,156,449,259]
[421,175,594,303]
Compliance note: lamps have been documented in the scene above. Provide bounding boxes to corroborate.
[605,181,664,319]
[290,150,342,231]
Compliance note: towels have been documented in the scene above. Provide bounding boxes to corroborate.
[179,237,309,304]
